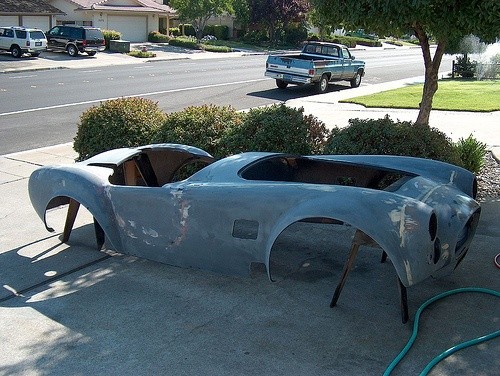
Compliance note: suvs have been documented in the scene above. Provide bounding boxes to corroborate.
[44,24,106,56]
[0,27,47,57]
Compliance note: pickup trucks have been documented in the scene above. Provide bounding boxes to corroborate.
[344,28,379,39]
[264,42,366,93]
